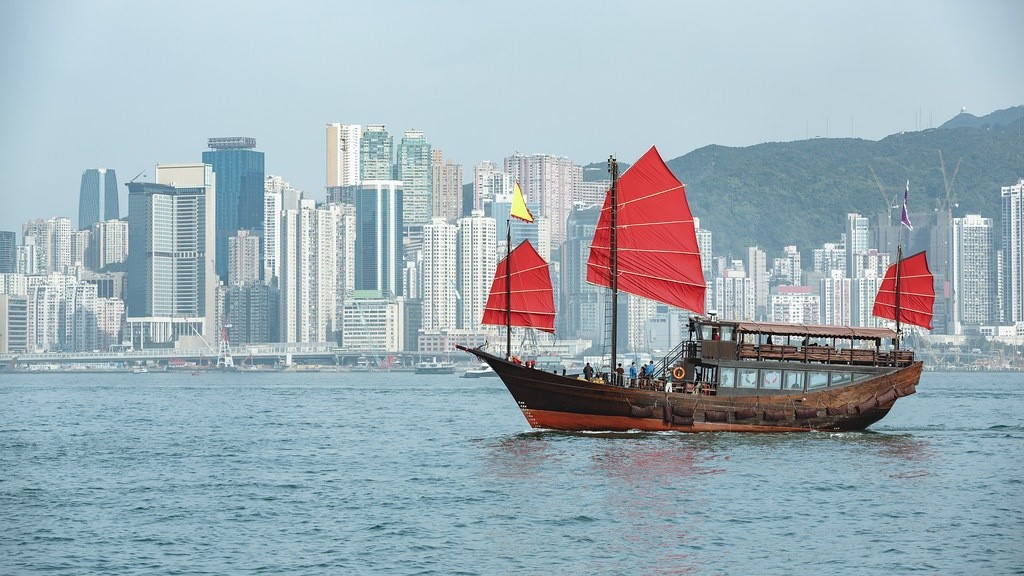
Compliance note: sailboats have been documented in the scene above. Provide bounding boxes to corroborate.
[453,143,936,433]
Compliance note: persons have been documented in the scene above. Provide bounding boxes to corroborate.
[713,330,719,340]
[694,369,701,385]
[638,360,655,389]
[616,363,624,385]
[583,363,594,380]
[630,362,637,387]
[665,369,673,393]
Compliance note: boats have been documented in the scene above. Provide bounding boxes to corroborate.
[133,368,148,374]
[459,363,499,378]
[415,362,456,374]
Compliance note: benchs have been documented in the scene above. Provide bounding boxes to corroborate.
[740,344,913,367]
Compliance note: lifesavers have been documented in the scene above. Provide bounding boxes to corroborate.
[673,367,685,379]
